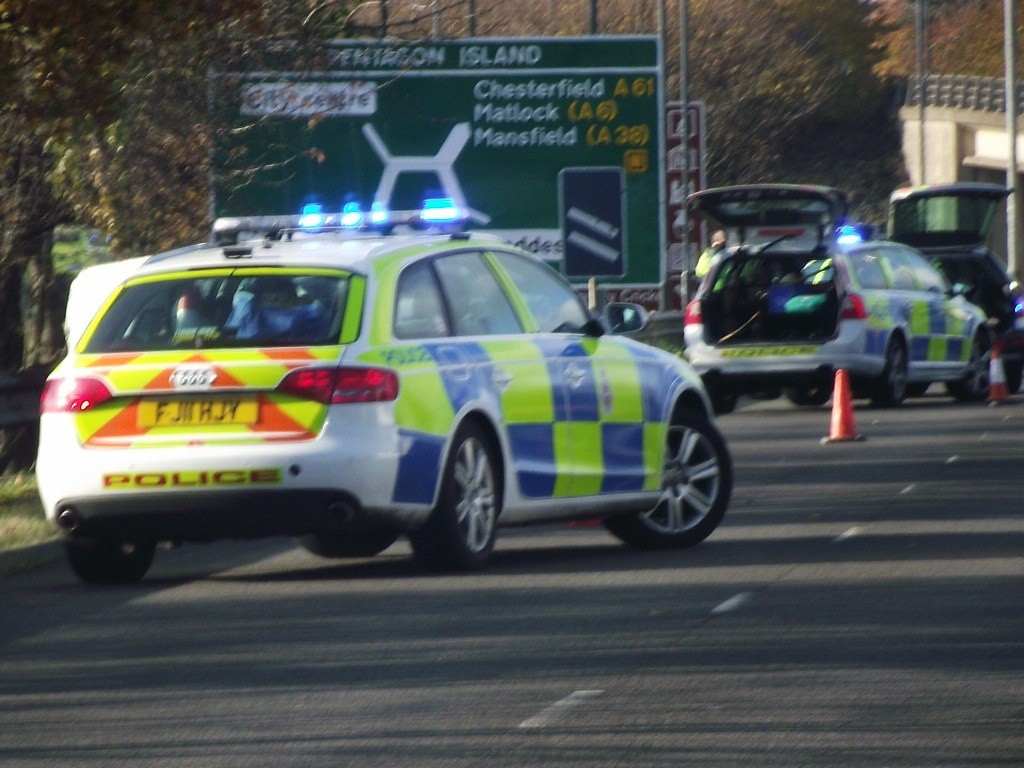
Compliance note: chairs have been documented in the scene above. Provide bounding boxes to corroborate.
[412,271,480,333]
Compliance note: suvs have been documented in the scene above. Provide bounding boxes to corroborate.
[677,181,993,417]
[36,203,738,585]
[883,180,1024,401]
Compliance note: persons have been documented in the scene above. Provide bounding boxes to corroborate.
[695,229,730,293]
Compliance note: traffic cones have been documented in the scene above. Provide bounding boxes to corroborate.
[822,367,880,441]
[984,345,1017,406]
